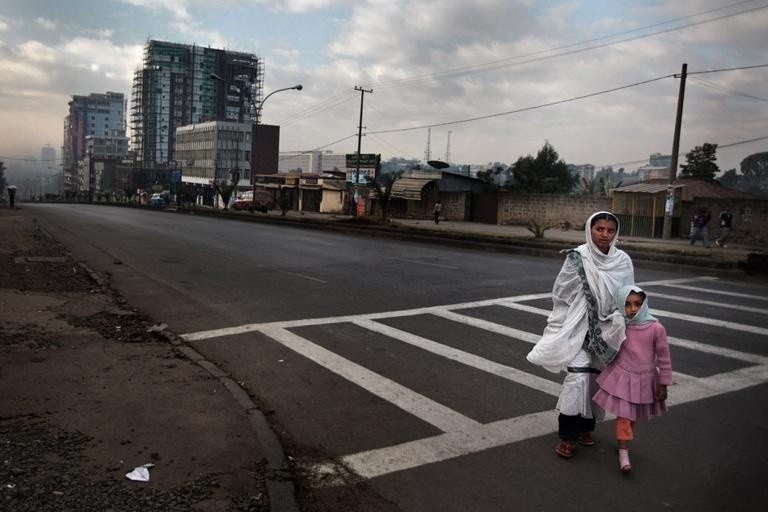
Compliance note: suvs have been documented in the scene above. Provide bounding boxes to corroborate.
[233,190,273,213]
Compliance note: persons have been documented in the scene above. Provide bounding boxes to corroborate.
[432,200,442,225]
[592,284,676,477]
[714,207,734,248]
[691,205,712,248]
[525,210,635,458]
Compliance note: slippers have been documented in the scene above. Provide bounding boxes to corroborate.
[577,434,594,445]
[556,439,575,457]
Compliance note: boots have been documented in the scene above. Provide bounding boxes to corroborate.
[619,448,632,472]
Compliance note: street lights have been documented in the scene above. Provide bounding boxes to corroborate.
[209,74,303,214]
